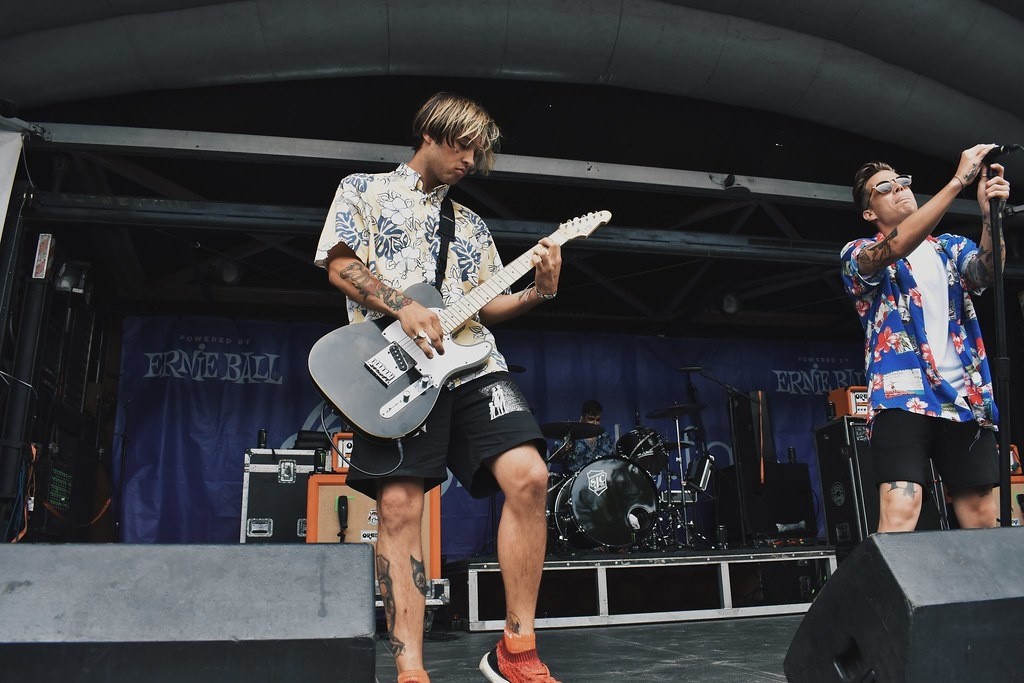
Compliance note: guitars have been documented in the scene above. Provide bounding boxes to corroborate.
[305,208,613,446]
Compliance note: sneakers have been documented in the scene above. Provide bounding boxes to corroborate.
[479,635,560,683]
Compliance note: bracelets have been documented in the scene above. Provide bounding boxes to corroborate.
[954,176,963,189]
[535,287,557,299]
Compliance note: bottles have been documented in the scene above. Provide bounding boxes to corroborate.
[258,429,268,449]
[314,448,332,474]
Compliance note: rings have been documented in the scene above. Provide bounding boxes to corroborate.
[413,331,425,342]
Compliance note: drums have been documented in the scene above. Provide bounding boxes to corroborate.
[546,424,669,558]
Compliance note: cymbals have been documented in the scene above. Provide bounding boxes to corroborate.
[645,402,711,422]
[661,441,692,448]
[540,421,605,440]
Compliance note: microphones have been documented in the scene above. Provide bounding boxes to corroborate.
[635,412,641,429]
[677,366,710,372]
[982,144,1021,163]
[338,496,348,531]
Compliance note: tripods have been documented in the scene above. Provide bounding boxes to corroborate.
[646,451,718,552]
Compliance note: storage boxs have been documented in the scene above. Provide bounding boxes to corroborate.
[240,448,317,545]
[812,414,952,548]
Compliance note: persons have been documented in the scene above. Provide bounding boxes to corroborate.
[314,92,562,683]
[568,400,615,473]
[840,144,1010,533]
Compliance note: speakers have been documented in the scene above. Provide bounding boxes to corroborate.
[814,415,949,552]
[0,543,378,683]
[775,462,817,538]
[782,526,1024,683]
[306,471,441,582]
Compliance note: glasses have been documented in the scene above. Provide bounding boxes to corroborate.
[864,174,912,211]
